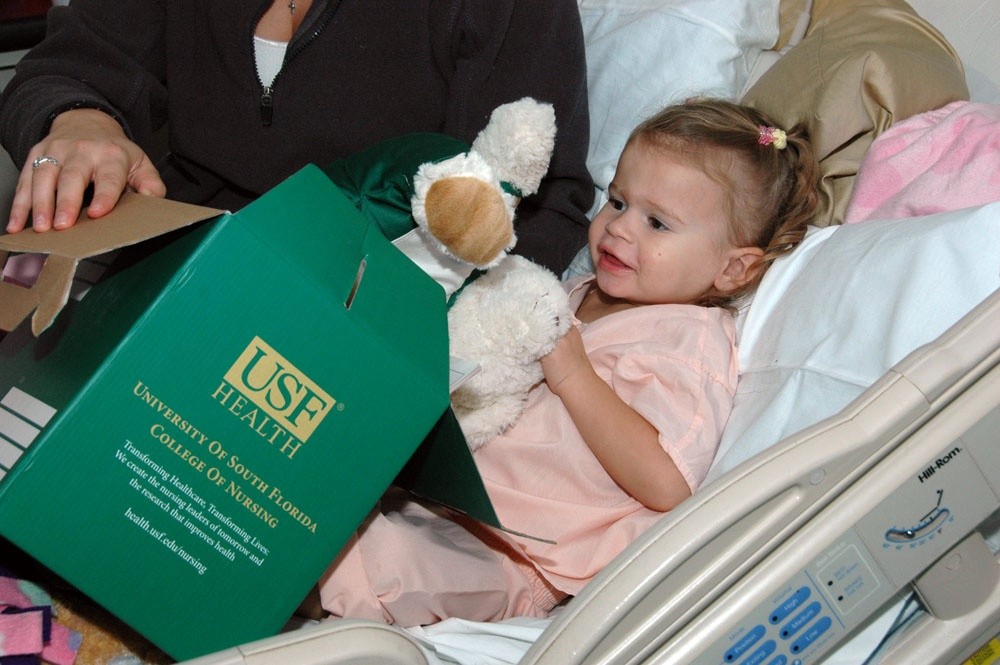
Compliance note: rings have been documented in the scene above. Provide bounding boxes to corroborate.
[32,156,64,171]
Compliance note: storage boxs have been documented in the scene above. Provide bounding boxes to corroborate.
[1,159,502,662]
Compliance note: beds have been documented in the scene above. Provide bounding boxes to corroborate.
[0,0,1000,665]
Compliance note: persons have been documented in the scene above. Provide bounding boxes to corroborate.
[293,97,821,627]
[0,0,597,282]
